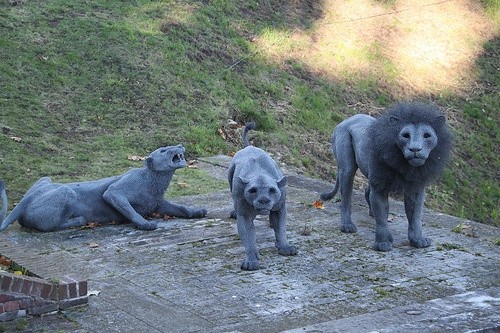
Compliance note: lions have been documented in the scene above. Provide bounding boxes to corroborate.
[320,100,452,251]
